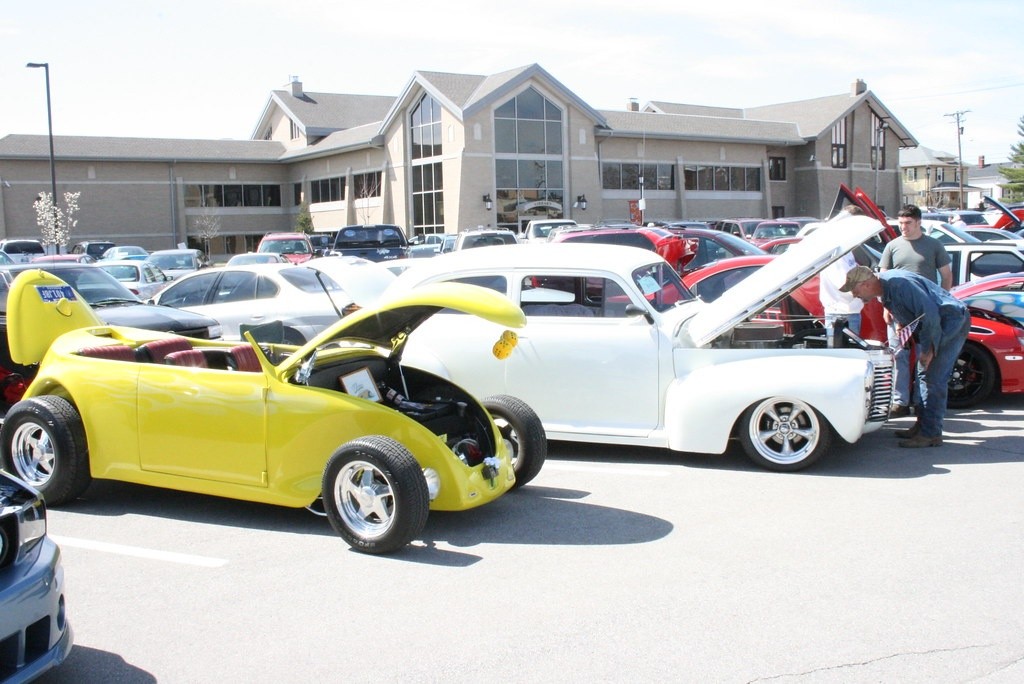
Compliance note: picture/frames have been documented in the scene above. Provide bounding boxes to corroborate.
[338,366,384,403]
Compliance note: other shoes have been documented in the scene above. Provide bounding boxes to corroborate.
[913,404,924,417]
[888,404,910,418]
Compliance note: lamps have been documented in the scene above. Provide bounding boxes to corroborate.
[578,194,588,210]
[483,193,492,210]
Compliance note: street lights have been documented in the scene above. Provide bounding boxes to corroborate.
[25,59,58,256]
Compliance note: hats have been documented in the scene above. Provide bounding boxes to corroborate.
[839,266,874,292]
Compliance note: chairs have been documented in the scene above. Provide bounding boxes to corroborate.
[226,345,262,372]
[164,350,208,369]
[78,346,144,362]
[139,337,193,364]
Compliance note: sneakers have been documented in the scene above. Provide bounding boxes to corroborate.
[895,417,921,439]
[899,432,943,448]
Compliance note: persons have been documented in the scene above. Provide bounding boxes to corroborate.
[839,266,971,448]
[878,205,952,419]
[819,204,871,337]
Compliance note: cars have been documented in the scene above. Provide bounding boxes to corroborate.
[0,468,74,684]
[0,184,1023,557]
